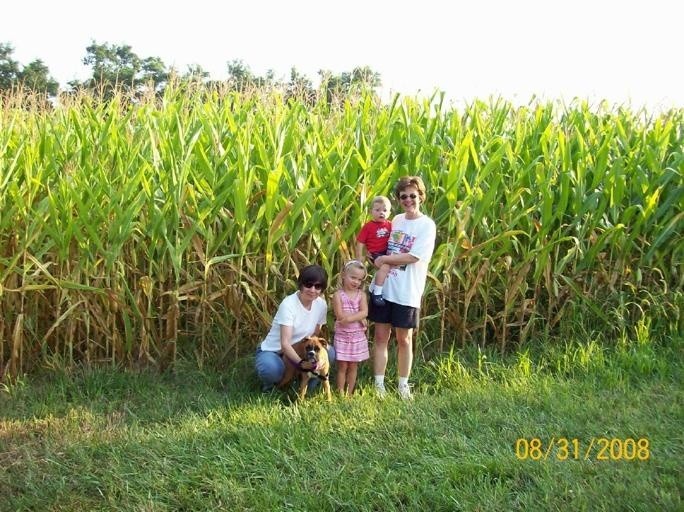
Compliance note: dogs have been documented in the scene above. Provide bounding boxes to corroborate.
[278,335,333,401]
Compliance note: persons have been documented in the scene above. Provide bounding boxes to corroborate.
[331,260,370,396]
[355,195,392,306]
[366,176,436,401]
[254,265,336,396]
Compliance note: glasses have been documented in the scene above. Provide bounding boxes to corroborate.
[302,282,322,290]
[399,194,418,199]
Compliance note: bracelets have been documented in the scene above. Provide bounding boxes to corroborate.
[377,256,383,265]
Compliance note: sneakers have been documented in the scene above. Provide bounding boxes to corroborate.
[259,383,272,392]
[377,383,386,392]
[397,384,410,398]
